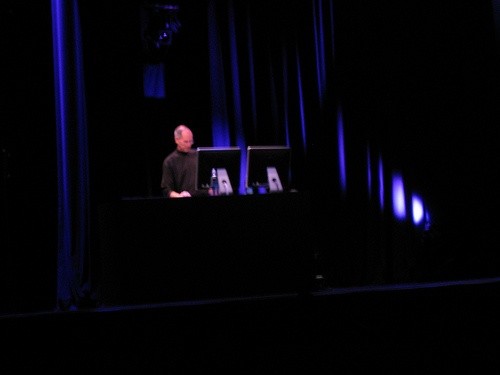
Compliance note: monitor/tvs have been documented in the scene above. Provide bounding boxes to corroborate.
[246,145,291,193]
[195,147,242,195]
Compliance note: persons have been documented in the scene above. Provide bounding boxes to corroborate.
[161,124,217,198]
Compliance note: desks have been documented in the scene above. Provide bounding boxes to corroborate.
[102,192,316,302]
